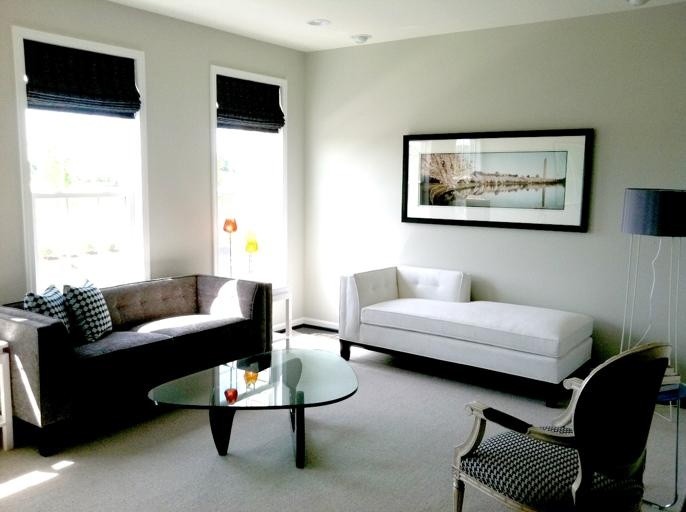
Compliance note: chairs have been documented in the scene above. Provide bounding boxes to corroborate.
[451,342,671,512]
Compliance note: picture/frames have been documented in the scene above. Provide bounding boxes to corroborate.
[402,128,596,233]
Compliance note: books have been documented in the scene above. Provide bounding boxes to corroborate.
[659,375,681,386]
[662,365,675,376]
[658,384,680,394]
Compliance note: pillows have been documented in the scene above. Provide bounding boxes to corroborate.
[63,280,114,343]
[23,284,70,336]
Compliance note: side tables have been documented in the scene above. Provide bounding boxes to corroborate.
[271,289,293,348]
[641,383,686,510]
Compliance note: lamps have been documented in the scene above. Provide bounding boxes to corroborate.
[619,188,686,392]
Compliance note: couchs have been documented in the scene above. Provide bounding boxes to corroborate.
[0,274,272,458]
[337,264,593,409]
